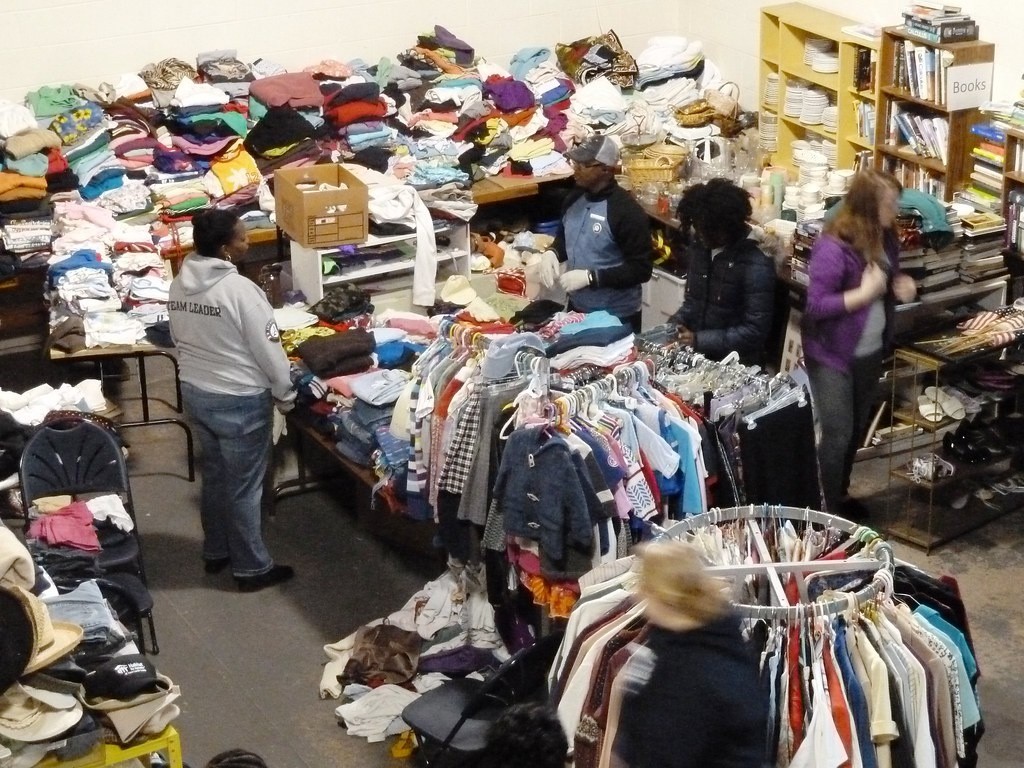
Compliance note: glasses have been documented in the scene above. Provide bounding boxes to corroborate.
[566,157,601,171]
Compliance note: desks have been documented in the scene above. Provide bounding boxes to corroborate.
[235,177,538,244]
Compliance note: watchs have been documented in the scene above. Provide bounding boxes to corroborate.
[587,271,593,285]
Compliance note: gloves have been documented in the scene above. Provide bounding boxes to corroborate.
[560,269,589,291]
[539,250,560,289]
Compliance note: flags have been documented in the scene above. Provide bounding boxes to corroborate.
[956,305,1024,347]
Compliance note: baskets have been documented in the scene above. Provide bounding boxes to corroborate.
[627,157,681,182]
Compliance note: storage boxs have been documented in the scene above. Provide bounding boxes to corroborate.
[642,267,689,335]
[275,163,368,249]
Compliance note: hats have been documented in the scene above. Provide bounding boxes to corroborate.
[563,133,622,168]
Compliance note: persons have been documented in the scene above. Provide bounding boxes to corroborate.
[538,135,654,339]
[483,702,568,768]
[611,540,768,768]
[168,207,297,593]
[799,168,914,522]
[669,178,780,373]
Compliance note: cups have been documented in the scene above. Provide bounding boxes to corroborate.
[801,186,825,204]
[783,186,801,205]
[826,169,855,190]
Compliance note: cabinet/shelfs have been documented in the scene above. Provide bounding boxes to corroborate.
[289,219,472,307]
[758,1,1024,558]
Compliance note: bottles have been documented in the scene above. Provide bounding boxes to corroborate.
[743,173,783,207]
[658,190,669,212]
[615,174,710,211]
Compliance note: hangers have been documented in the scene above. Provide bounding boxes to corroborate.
[411,318,810,441]
[625,502,922,666]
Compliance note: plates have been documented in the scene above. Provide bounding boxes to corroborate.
[784,79,837,133]
[764,73,779,104]
[782,201,826,225]
[800,169,830,186]
[790,127,837,169]
[759,109,777,152]
[803,36,838,73]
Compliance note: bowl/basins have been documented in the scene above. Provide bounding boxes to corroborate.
[762,167,786,180]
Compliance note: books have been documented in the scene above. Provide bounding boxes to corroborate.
[882,155,946,202]
[895,198,1011,294]
[841,20,896,42]
[789,218,825,284]
[1015,137,1024,172]
[852,45,877,172]
[1008,187,1024,255]
[954,120,1006,215]
[902,0,979,44]
[978,100,1024,132]
[884,96,950,167]
[892,39,953,107]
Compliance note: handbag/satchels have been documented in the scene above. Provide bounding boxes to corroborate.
[669,101,717,127]
[705,81,743,136]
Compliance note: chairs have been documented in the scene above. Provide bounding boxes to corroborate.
[402,630,566,768]
[17,416,160,655]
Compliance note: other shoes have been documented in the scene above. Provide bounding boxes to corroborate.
[237,563,293,593]
[204,557,230,574]
[906,356,1023,511]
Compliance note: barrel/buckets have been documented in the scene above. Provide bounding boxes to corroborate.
[531,187,576,263]
